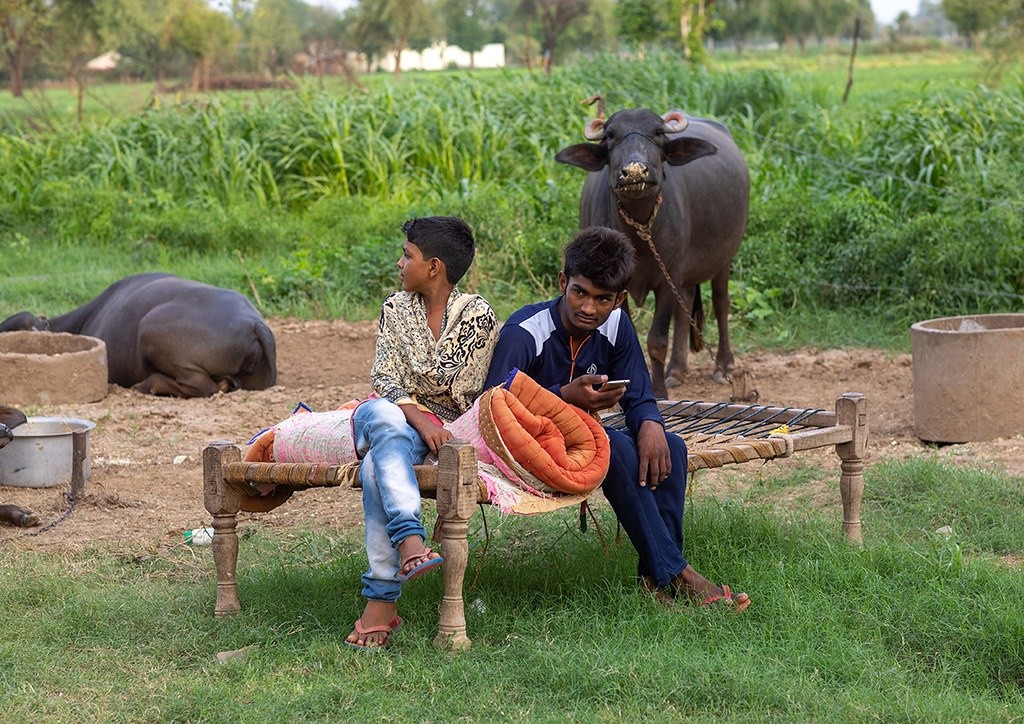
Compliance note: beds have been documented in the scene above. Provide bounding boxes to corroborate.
[204,388,868,652]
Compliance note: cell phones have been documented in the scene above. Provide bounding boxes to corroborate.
[598,380,630,393]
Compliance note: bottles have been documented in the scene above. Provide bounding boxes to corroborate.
[185,528,213,545]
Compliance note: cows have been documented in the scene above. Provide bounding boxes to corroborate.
[0,405,45,528]
[0,272,278,400]
[554,95,749,402]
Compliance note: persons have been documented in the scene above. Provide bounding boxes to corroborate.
[482,226,751,613]
[344,216,500,651]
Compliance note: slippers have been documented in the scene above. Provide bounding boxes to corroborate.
[397,548,444,581]
[694,585,751,612]
[344,615,404,651]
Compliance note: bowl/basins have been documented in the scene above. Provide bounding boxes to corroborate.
[0,417,95,487]
